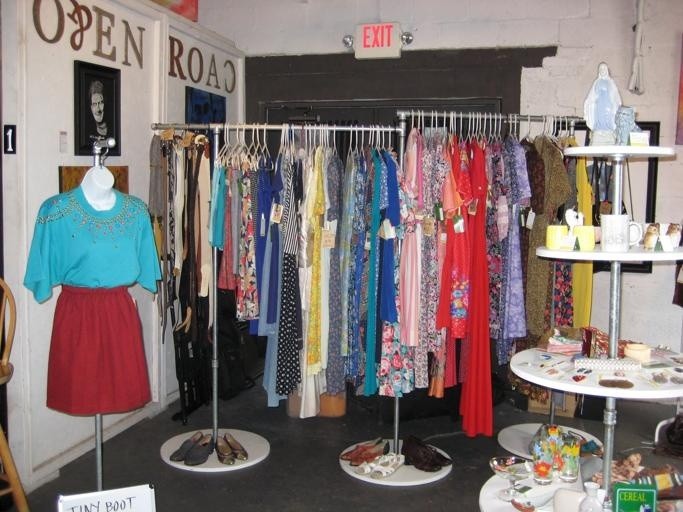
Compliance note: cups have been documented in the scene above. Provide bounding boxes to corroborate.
[573,225,595,252]
[541,423,563,472]
[546,225,568,251]
[534,436,553,486]
[601,213,643,253]
[558,436,580,483]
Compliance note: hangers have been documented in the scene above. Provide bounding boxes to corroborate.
[408,107,579,149]
[155,121,400,170]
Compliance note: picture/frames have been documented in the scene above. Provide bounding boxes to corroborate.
[549,120,662,273]
[74,59,121,156]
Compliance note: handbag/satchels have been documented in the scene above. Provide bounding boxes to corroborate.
[592,201,628,243]
[197,316,254,401]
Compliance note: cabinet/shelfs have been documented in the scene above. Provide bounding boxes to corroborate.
[479,107,683,511]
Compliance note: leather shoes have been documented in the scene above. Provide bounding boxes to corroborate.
[402,436,442,472]
[341,437,382,460]
[224,433,248,461]
[169,431,203,461]
[184,434,214,466]
[215,436,235,464]
[401,434,453,466]
[350,440,390,466]
[528,424,548,455]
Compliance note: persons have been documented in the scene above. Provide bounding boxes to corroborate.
[82,78,111,147]
[189,92,212,156]
[579,62,624,133]
[22,164,164,418]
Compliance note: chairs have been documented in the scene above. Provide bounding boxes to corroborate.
[0,278,30,512]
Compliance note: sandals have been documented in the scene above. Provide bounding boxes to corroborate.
[568,430,602,455]
[370,454,405,479]
[355,452,397,475]
[561,432,593,458]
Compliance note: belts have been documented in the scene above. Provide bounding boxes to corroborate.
[168,139,175,306]
[187,132,215,344]
[171,137,184,335]
[173,147,184,324]
[160,140,169,344]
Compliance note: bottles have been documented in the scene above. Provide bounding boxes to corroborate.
[579,480,603,511]
